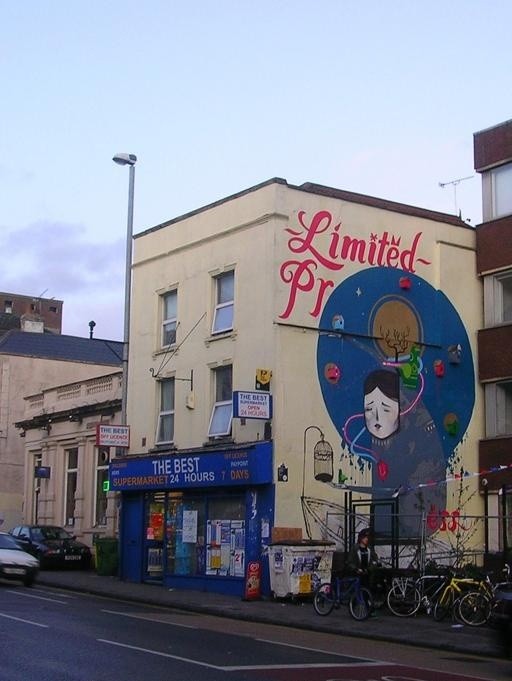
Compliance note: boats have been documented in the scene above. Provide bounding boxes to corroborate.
[301,495,463,569]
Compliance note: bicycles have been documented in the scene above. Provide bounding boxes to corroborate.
[312,564,374,621]
[376,561,512,630]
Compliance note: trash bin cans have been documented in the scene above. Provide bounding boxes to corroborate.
[94,537,118,576]
[267,539,336,604]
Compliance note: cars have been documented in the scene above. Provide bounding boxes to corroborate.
[0,532,41,587]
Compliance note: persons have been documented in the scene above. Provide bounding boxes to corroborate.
[343,528,381,621]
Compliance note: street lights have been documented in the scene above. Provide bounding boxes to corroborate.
[113,153,138,456]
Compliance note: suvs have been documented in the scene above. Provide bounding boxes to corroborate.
[8,525,91,570]
